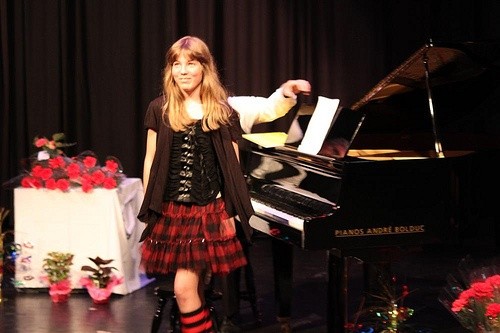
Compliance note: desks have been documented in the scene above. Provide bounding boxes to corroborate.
[14,178,157,295]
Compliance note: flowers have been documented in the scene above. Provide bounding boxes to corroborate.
[20,133,119,193]
[451,274,500,319]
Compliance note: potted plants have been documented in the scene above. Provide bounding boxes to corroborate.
[80,256,122,305]
[42,252,74,303]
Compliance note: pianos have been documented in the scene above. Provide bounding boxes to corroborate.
[235,39,479,333]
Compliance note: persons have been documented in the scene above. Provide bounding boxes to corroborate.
[319,138,350,157]
[137,36,255,333]
[226,78,311,175]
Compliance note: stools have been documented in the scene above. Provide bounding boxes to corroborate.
[150,287,223,333]
